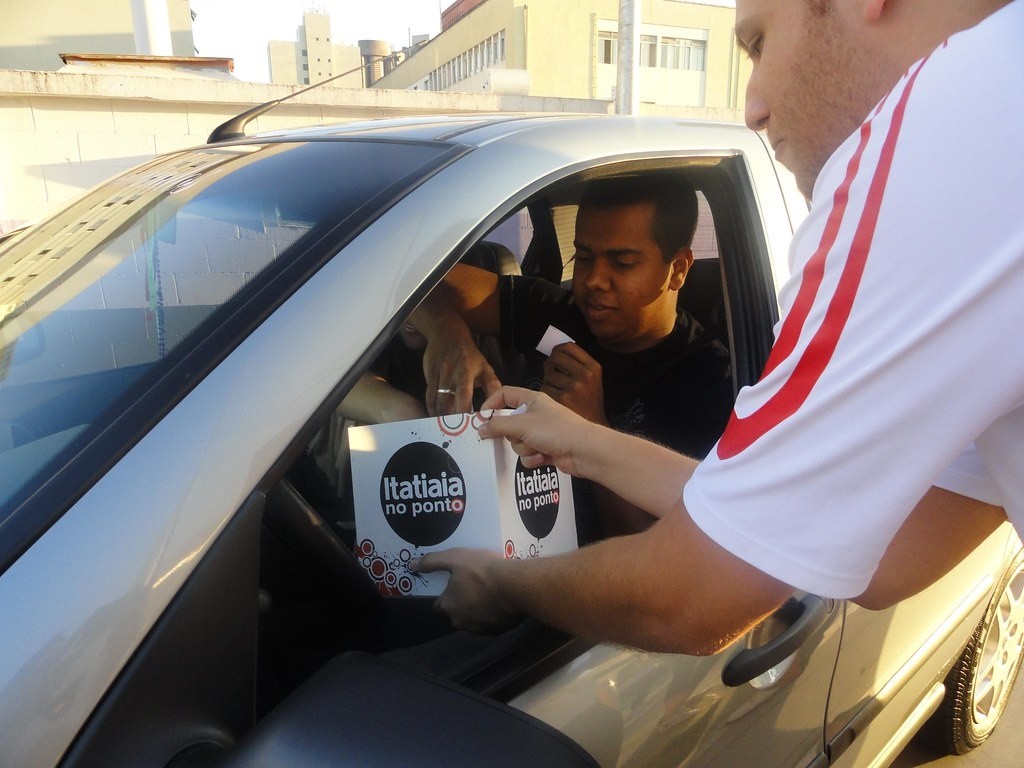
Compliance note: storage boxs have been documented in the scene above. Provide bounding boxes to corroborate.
[348,410,577,597]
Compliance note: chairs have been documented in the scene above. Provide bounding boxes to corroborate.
[458,242,524,389]
[535,257,730,353]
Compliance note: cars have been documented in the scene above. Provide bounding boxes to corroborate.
[6,112,1024,762]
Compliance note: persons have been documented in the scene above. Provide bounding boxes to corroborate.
[306,323,428,527]
[403,1,1024,656]
[403,179,736,708]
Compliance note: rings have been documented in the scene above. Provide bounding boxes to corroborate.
[435,388,455,395]
